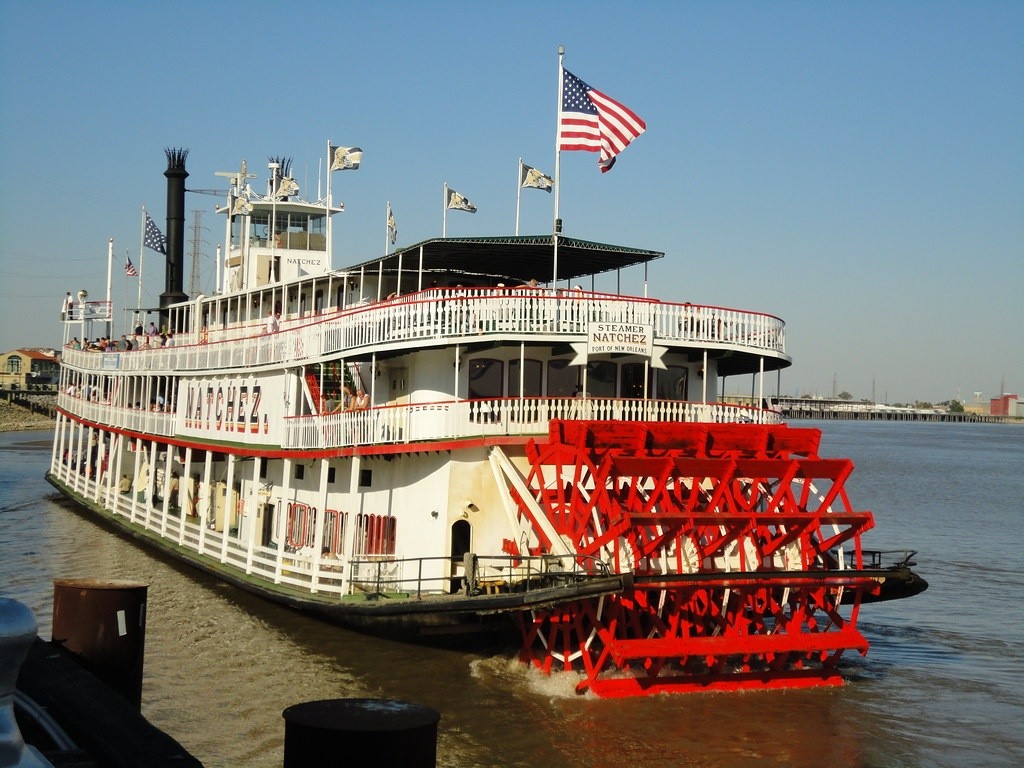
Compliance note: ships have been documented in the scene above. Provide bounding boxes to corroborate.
[42,44,930,703]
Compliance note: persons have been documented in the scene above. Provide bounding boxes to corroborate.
[136,324,143,334]
[531,279,538,287]
[170,472,180,508]
[67,379,112,402]
[82,335,138,351]
[72,337,81,350]
[322,546,339,559]
[67,292,73,319]
[128,388,177,413]
[266,311,280,332]
[62,449,68,465]
[121,474,131,492]
[148,322,157,336]
[160,333,175,347]
[321,383,376,415]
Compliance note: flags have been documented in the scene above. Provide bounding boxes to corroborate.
[329,145,362,171]
[231,195,254,223]
[275,174,299,201]
[125,256,138,276]
[388,209,397,244]
[446,188,477,213]
[144,212,166,255]
[521,163,554,193]
[559,68,646,173]
